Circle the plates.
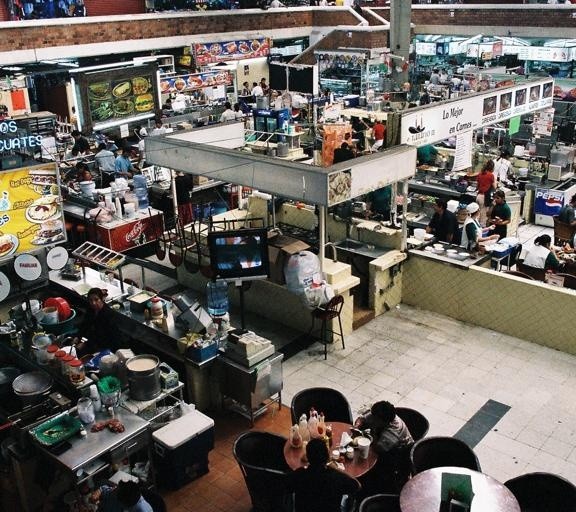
[188,78,202,87]
[446,249,458,254]
[0,271,11,303]
[12,253,43,281]
[26,201,61,223]
[239,44,250,54]
[47,247,68,271]
[458,252,470,257]
[174,78,186,92]
[114,101,134,114]
[250,42,261,51]
[113,80,131,98]
[0,234,19,260]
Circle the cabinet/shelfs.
[336,67,361,94]
[29,397,150,511]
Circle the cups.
[105,195,111,206]
[358,437,371,460]
[123,301,131,314]
[124,202,136,221]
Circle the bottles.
[338,448,346,460]
[308,408,318,438]
[115,197,122,218]
[63,353,76,377]
[77,397,95,423]
[151,297,164,323]
[316,413,325,437]
[289,424,302,448]
[298,413,309,441]
[321,435,329,453]
[332,450,339,460]
[326,426,334,447]
[47,345,61,367]
[144,309,149,321]
[346,447,354,460]
[161,316,168,333]
[89,384,102,412]
[54,350,64,371]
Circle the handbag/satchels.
[299,277,339,311]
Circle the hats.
[435,198,447,210]
[466,202,479,217]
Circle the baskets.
[34,414,82,444]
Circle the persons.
[344,133,357,153]
[136,123,152,169]
[93,144,115,188]
[426,199,459,243]
[71,130,90,157]
[117,136,127,149]
[561,230,576,254]
[162,98,173,110]
[175,169,193,226]
[333,142,355,164]
[348,401,416,494]
[371,119,385,153]
[191,88,199,105]
[461,202,501,250]
[352,119,370,152]
[60,162,97,201]
[472,159,497,228]
[269,88,283,110]
[514,142,526,158]
[489,150,515,187]
[70,288,122,353]
[241,81,250,95]
[217,102,236,122]
[232,102,244,119]
[486,190,510,241]
[250,83,264,103]
[521,235,559,282]
[0,104,9,121]
[287,438,362,512]
[106,140,118,151]
[150,119,173,136]
[427,68,490,92]
[232,237,261,269]
[192,88,209,105]
[98,480,153,511]
[259,78,269,95]
[113,148,139,180]
[468,57,491,68]
[366,185,391,221]
[398,83,419,102]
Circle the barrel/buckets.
[12,372,53,405]
[519,168,528,177]
[205,279,230,316]
[41,306,59,324]
[133,176,148,201]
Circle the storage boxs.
[152,409,215,469]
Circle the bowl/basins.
[434,248,445,254]
[414,229,426,241]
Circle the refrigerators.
[253,108,290,143]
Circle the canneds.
[47,345,58,369]
[62,355,75,376]
[54,351,66,373]
[150,297,163,318]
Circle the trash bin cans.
[152,409,215,491]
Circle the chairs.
[232,431,291,512]
[504,472,576,512]
[291,387,353,426]
[310,295,344,360]
[283,491,358,511]
[410,436,481,476]
[359,494,402,512]
[502,215,576,290]
[147,207,235,279]
[396,407,429,440]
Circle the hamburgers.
[134,93,154,113]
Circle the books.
[439,472,475,512]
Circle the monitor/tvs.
[207,228,271,287]
